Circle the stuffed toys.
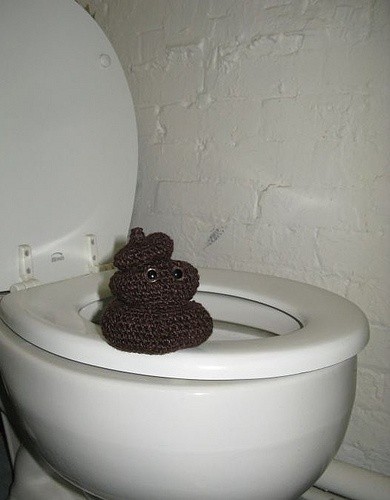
[102,224,214,357]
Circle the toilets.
[1,1,369,500]
